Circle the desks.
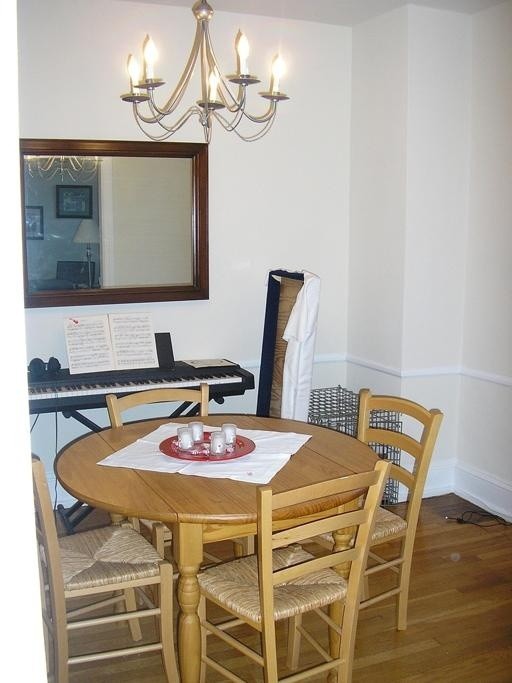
[54,413,384,683]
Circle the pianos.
[28,359,255,414]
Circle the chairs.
[197,458,393,683]
[296,388,443,630]
[105,381,256,581]
[31,452,182,683]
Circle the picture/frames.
[24,206,44,240]
[55,261,95,287]
[56,184,93,219]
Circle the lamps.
[120,2,289,144]
[24,155,101,184]
[72,219,100,289]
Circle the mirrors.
[19,137,210,309]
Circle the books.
[64,311,159,375]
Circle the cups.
[209,430,227,455]
[188,421,204,443]
[177,425,194,449]
[221,423,237,448]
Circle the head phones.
[28,356,61,378]
